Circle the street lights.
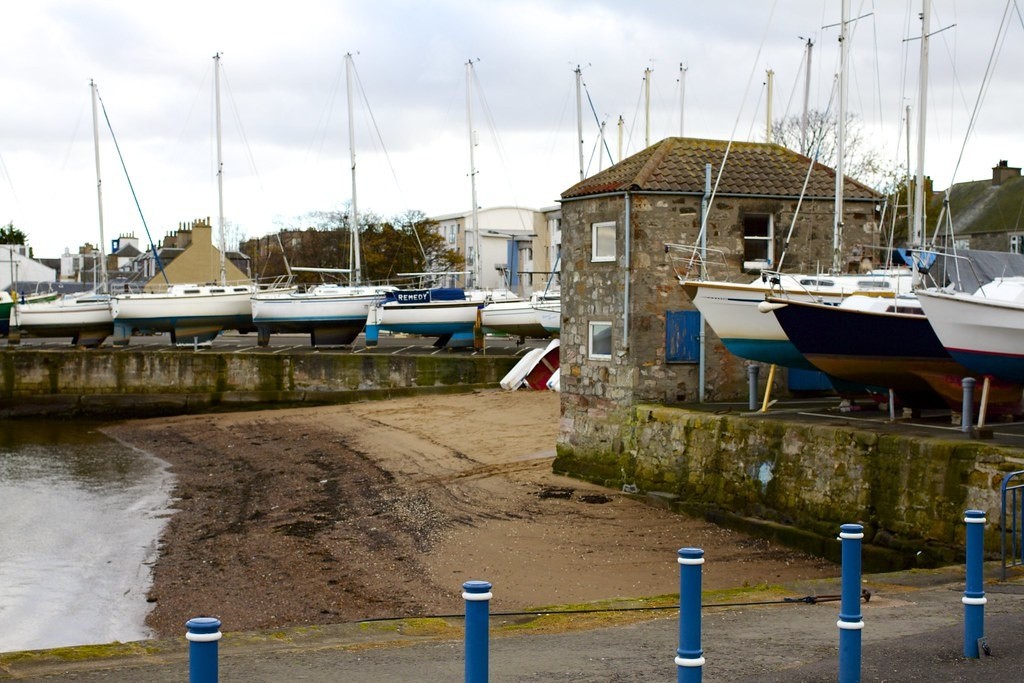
[488,230,538,291]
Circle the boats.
[500,338,560,392]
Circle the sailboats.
[109,51,297,347]
[663,0,1024,415]
[0,155,57,337]
[8,78,171,349]
[250,52,437,347]
[481,63,653,338]
[366,58,530,347]
